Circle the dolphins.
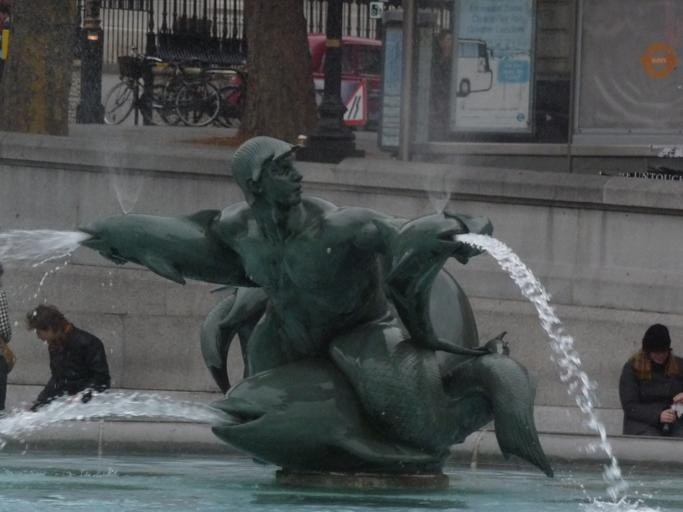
[78,212,264,289]
[209,360,494,467]
[384,212,512,355]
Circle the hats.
[235,32,383,127]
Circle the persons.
[24,305,111,410]
[619,324,683,436]
[0,267,12,416]
[211,135,403,373]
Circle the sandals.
[457,37,494,96]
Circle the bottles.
[641,324,674,353]
